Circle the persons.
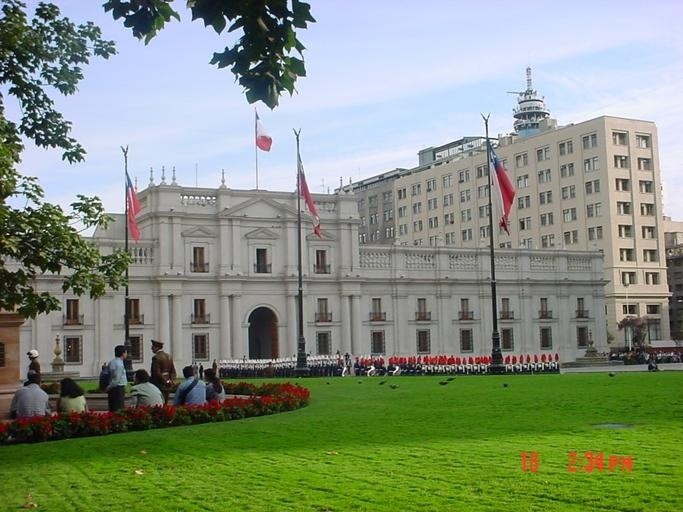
[26,349,39,381]
[150,338,181,407]
[212,360,217,376]
[131,369,165,410]
[10,373,51,419]
[191,361,195,374]
[56,377,88,421]
[612,349,682,363]
[23,369,36,387]
[199,363,204,379]
[194,362,199,380]
[105,344,129,413]
[202,368,225,403]
[174,366,207,406]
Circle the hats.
[151,339,163,347]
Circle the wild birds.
[380,379,386,385]
[503,383,508,387]
[438,381,448,385]
[357,380,363,384]
[447,377,456,381]
[609,372,615,377]
[389,383,399,388]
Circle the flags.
[290,126,323,241]
[479,110,515,239]
[120,144,142,248]
[254,108,273,154]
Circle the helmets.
[27,350,39,358]
